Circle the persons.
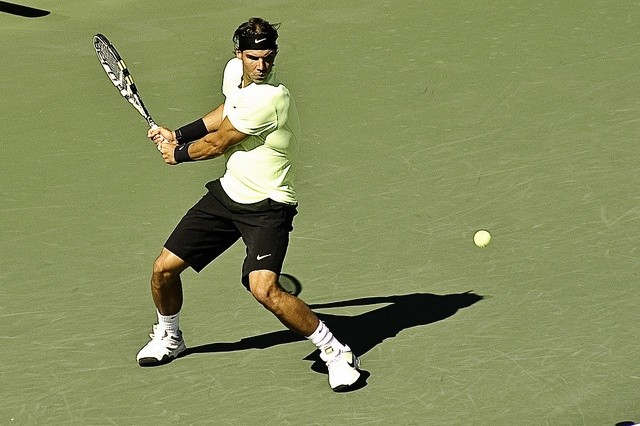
[135,16,363,392]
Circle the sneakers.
[319,344,361,392]
[136,325,188,367]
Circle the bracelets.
[175,118,209,141]
[172,142,194,167]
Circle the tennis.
[474,229,492,249]
